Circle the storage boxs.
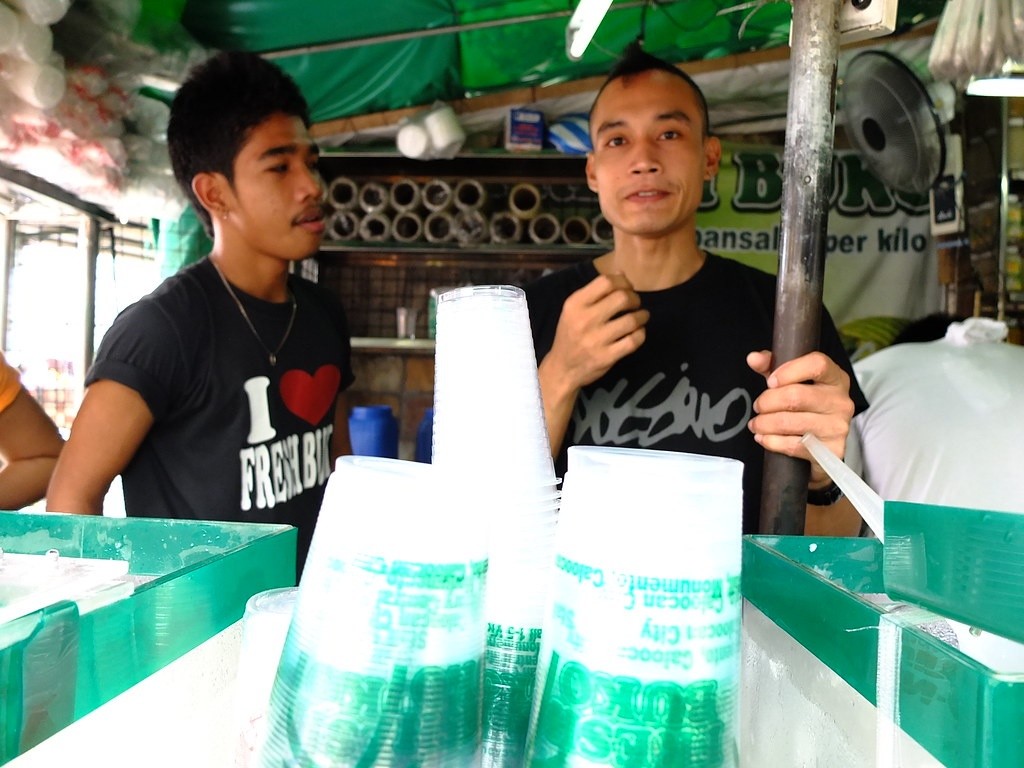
[741,499,1024,768]
[0,508,297,768]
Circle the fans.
[840,49,966,239]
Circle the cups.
[0,1,75,107]
[426,107,465,151]
[397,126,431,161]
[235,287,743,767]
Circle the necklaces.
[209,253,296,365]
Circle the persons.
[0,349,65,510]
[45,49,355,586]
[527,54,868,536]
[834,310,1024,531]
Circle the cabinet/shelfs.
[309,151,618,354]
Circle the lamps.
[564,0,615,62]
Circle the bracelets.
[806,459,845,506]
